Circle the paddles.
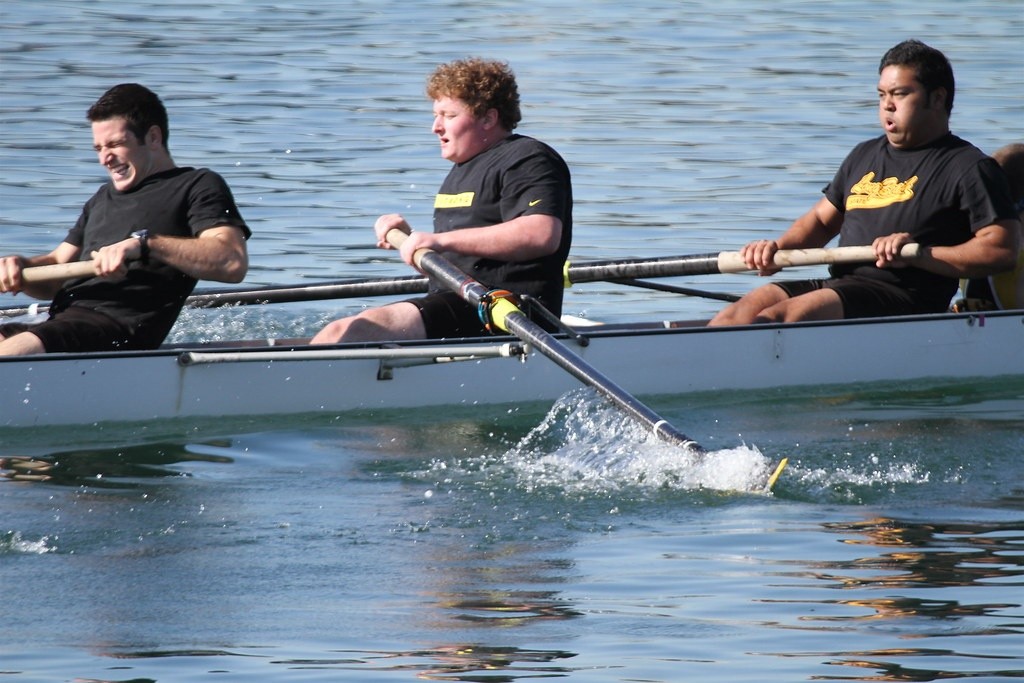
[4,258,97,284]
[0,243,920,322]
[384,225,790,494]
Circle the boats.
[1,296,1024,430]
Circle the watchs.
[131,229,149,260]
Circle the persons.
[0,83,252,359]
[309,56,572,350]
[704,38,1023,328]
